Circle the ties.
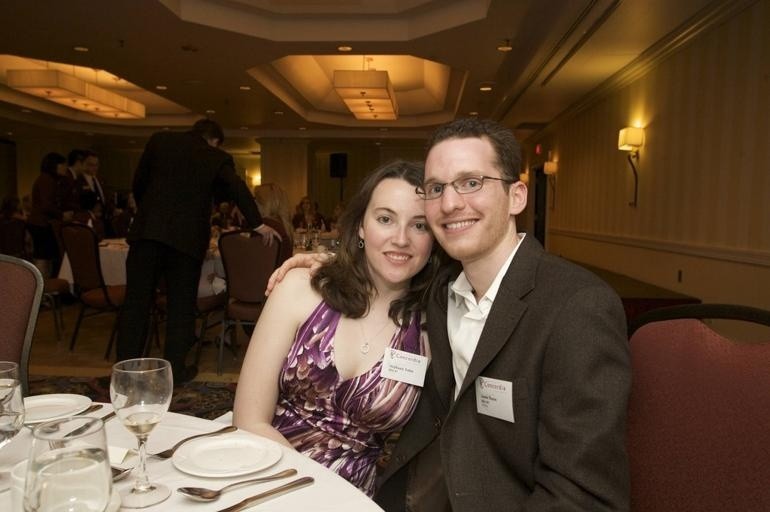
[91,177,103,207]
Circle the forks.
[131,425,239,461]
[46,438,68,450]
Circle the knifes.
[53,409,117,446]
[216,476,315,512]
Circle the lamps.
[618,127,647,207]
[334,55,401,121]
[5,61,146,120]
[543,161,561,212]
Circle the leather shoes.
[172,365,198,377]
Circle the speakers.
[327,151,347,178]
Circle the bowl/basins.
[8,459,25,510]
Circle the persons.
[113,190,127,230]
[231,157,450,497]
[259,114,635,511]
[291,197,326,235]
[2,195,27,224]
[125,192,138,238]
[240,183,295,270]
[210,202,237,230]
[63,147,90,237]
[112,116,284,388]
[78,152,122,243]
[320,201,349,241]
[27,152,74,310]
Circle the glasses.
[415,174,513,200]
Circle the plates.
[1,487,122,512]
[23,393,92,424]
[172,435,282,478]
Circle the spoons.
[113,468,135,482]
[175,468,298,503]
[36,404,103,434]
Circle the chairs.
[218,229,281,330]
[0,254,44,395]
[43,278,70,341]
[145,279,239,368]
[61,222,127,360]
[627,302,770,511]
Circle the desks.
[561,255,704,320]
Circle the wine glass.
[22,417,113,511]
[109,357,171,510]
[1,359,25,496]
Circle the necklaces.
[360,316,391,353]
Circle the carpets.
[29,374,237,422]
